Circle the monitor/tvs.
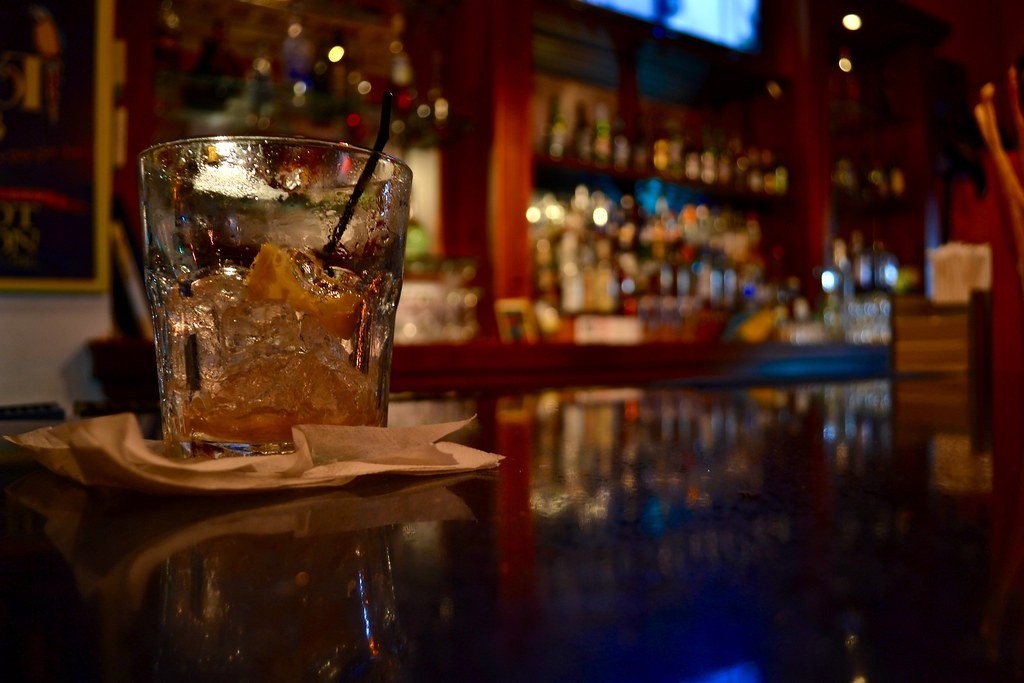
[571,0,768,61]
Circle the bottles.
[527,380,887,496]
[833,232,900,299]
[541,200,791,314]
[536,96,787,192]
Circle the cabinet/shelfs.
[442,0,930,338]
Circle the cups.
[158,526,402,683]
[140,136,416,462]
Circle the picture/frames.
[0,0,116,294]
